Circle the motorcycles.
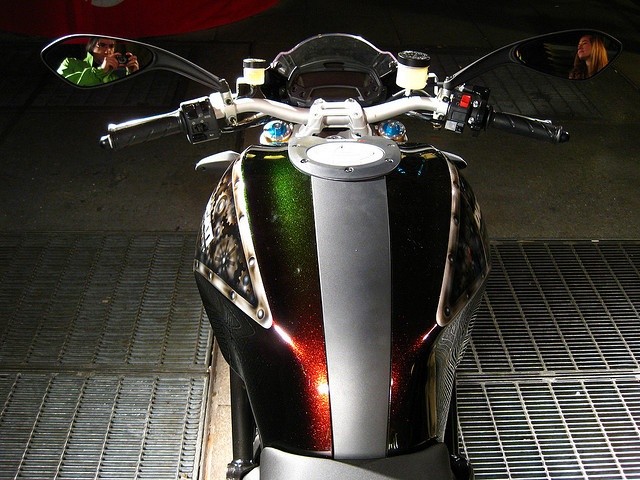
[37,27,625,480]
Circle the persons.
[56,36,140,88]
[571,33,609,81]
